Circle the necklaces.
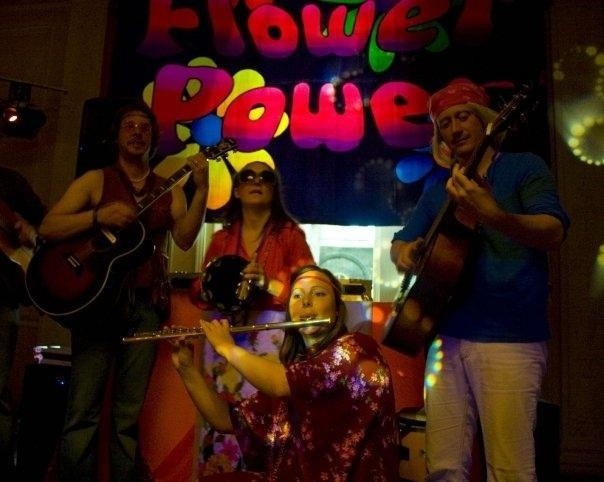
[114,167,150,183]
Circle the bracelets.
[92,206,97,226]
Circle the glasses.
[120,119,153,133]
[235,168,277,184]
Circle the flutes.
[121,318,332,344]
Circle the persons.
[199,160,315,482]
[162,265,401,482]
[390,77,570,482]
[39,109,208,482]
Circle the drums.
[397,404,483,482]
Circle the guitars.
[24,136,238,319]
[381,88,535,359]
